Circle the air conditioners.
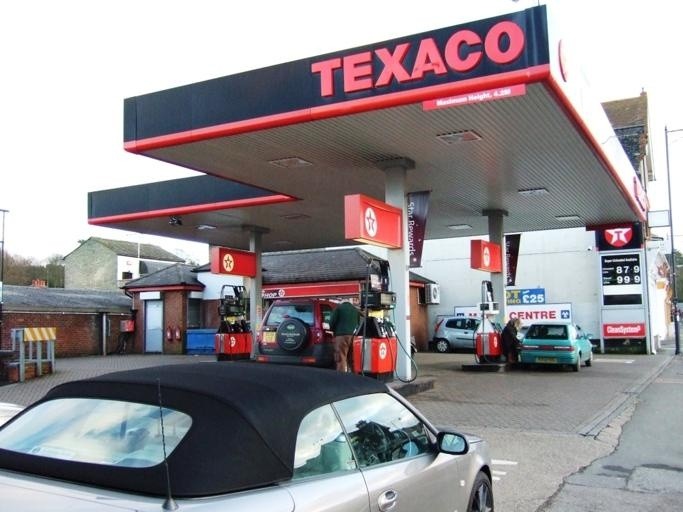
[423,283,441,304]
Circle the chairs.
[537,327,548,338]
[450,321,457,327]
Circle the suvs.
[252,298,365,368]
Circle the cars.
[0,362,495,511]
[433,317,496,353]
[517,322,595,372]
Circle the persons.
[499,318,521,362]
[328,297,361,373]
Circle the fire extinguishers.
[165,325,181,340]
